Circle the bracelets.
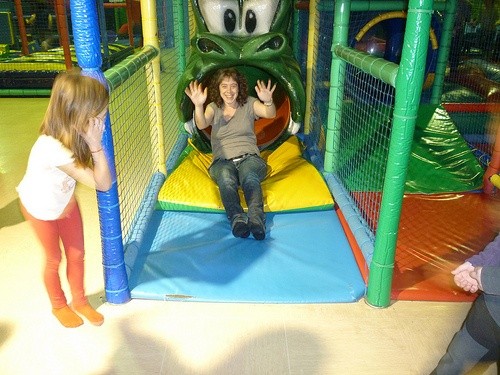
[91,149,102,154]
[264,98,272,105]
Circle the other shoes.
[231,217,250,238]
[248,214,265,241]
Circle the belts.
[229,155,242,160]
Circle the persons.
[184,68,276,240]
[26,36,40,52]
[119,20,135,37]
[430,234,500,375]
[17,73,112,327]
